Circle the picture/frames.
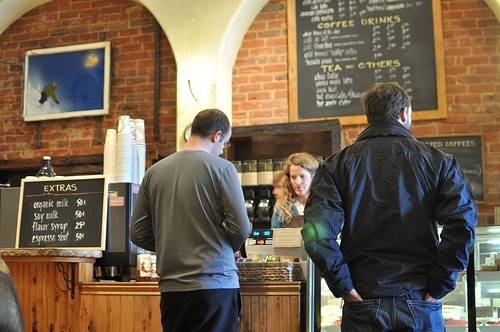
[23,41,111,122]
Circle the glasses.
[221,133,231,149]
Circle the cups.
[103,115,146,191]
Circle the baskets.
[236,261,302,282]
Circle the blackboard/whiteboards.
[414,133,486,204]
[286,0,449,126]
[14,174,110,250]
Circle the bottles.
[35,155,57,177]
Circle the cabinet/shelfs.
[314,224,500,332]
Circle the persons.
[271,152,319,229]
[39,82,61,105]
[300,82,478,332]
[130,109,250,332]
